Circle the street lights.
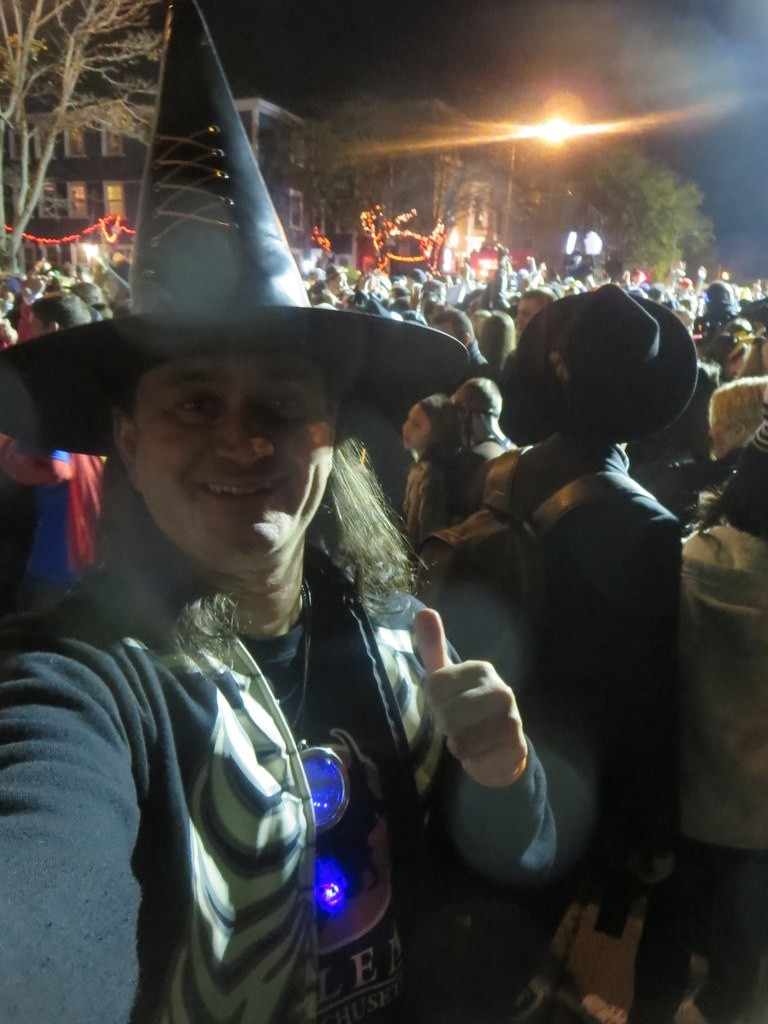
[503,112,578,242]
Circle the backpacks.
[415,447,658,702]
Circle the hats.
[407,265,425,284]
[0,0,469,456]
[515,284,698,443]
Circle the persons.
[0,245,768,661]
[676,419,768,1024]
[416,283,685,991]
[1,193,558,1024]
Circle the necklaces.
[268,582,351,832]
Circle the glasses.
[298,744,352,927]
